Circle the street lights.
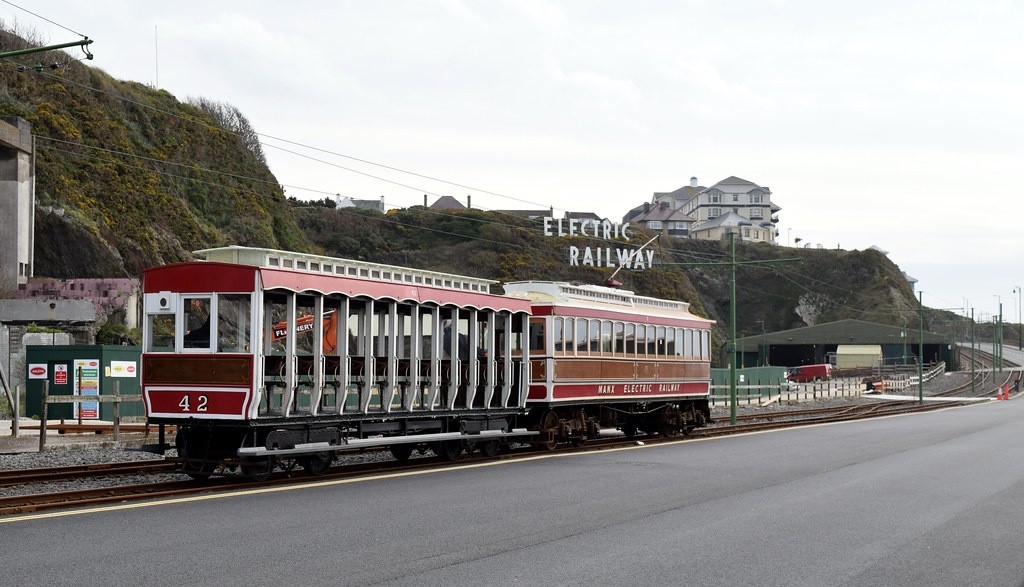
[788,227,791,247]
[728,221,732,232]
[1013,286,1022,350]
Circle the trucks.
[787,364,832,383]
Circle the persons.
[171,313,210,348]
[444,320,488,360]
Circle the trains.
[140,261,716,481]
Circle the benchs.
[259,355,522,413]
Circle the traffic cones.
[997,383,1009,400]
